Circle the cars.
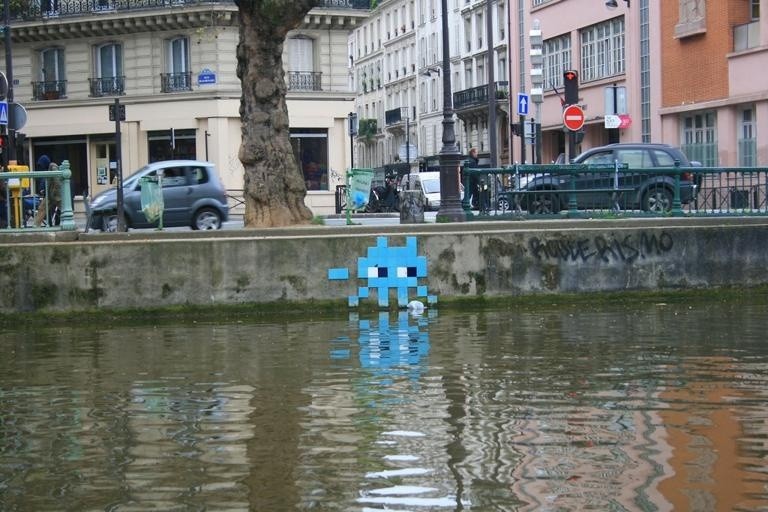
[497,144,703,214]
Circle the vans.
[88,160,229,232]
[399,170,473,210]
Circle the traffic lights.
[563,70,578,104]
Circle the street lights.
[529,19,543,163]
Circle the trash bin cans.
[351,166,376,211]
[138,180,164,226]
[731,190,748,209]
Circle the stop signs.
[563,105,584,131]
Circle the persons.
[462,148,480,211]
[38,153,63,227]
